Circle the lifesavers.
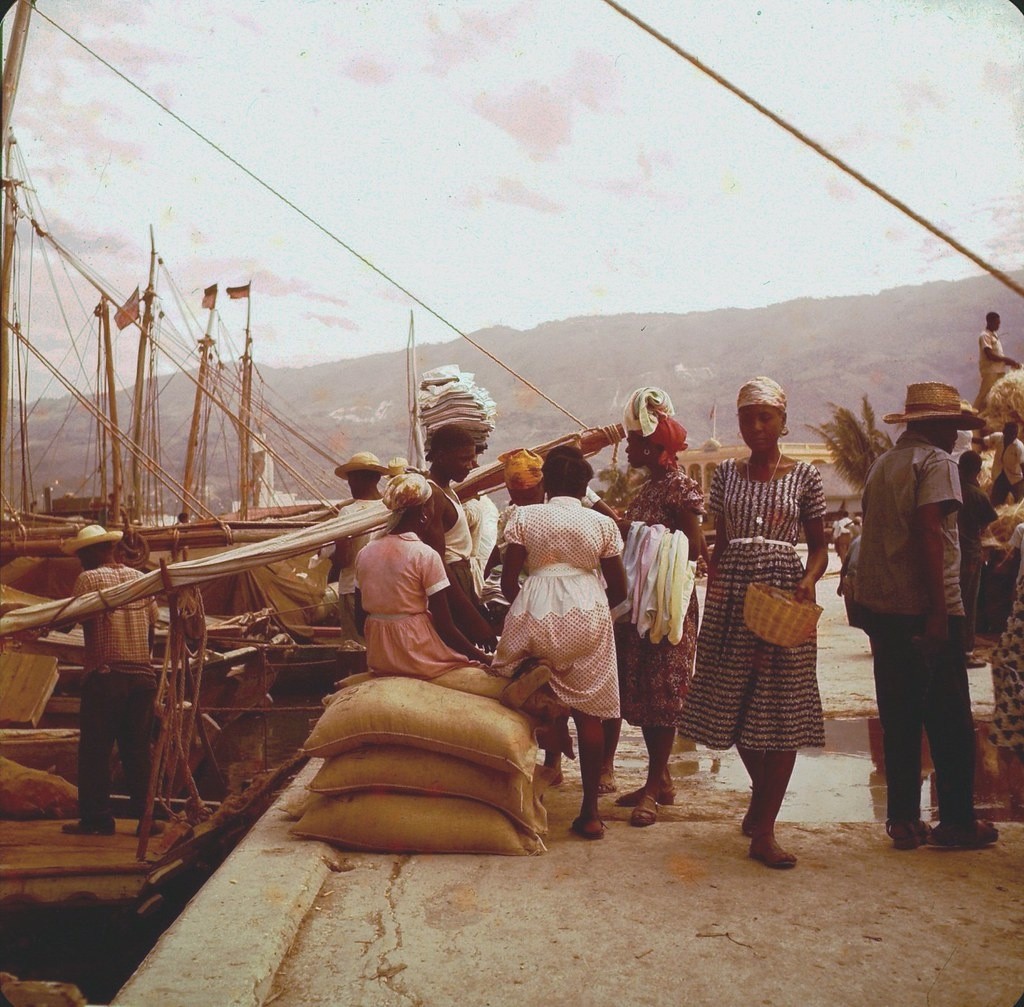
[1,893,38,911]
[62,891,97,909]
[115,532,150,569]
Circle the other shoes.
[63,822,114,835]
[500,657,552,710]
[965,655,986,668]
[749,847,796,867]
[136,822,165,838]
[743,789,760,837]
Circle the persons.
[675,376,829,870]
[837,311,1024,848]
[0,524,167,836]
[335,414,703,839]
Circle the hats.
[498,447,544,489]
[334,452,390,480]
[737,377,788,413]
[882,383,986,431]
[58,525,123,556]
[426,425,476,461]
[388,457,408,475]
[373,473,432,540]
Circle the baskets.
[744,583,824,647]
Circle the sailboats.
[0,0,373,1006]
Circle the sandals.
[886,818,932,850]
[927,820,998,847]
[597,768,616,793]
[631,794,657,824]
[573,816,604,840]
[615,786,677,805]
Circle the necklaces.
[746,455,781,524]
